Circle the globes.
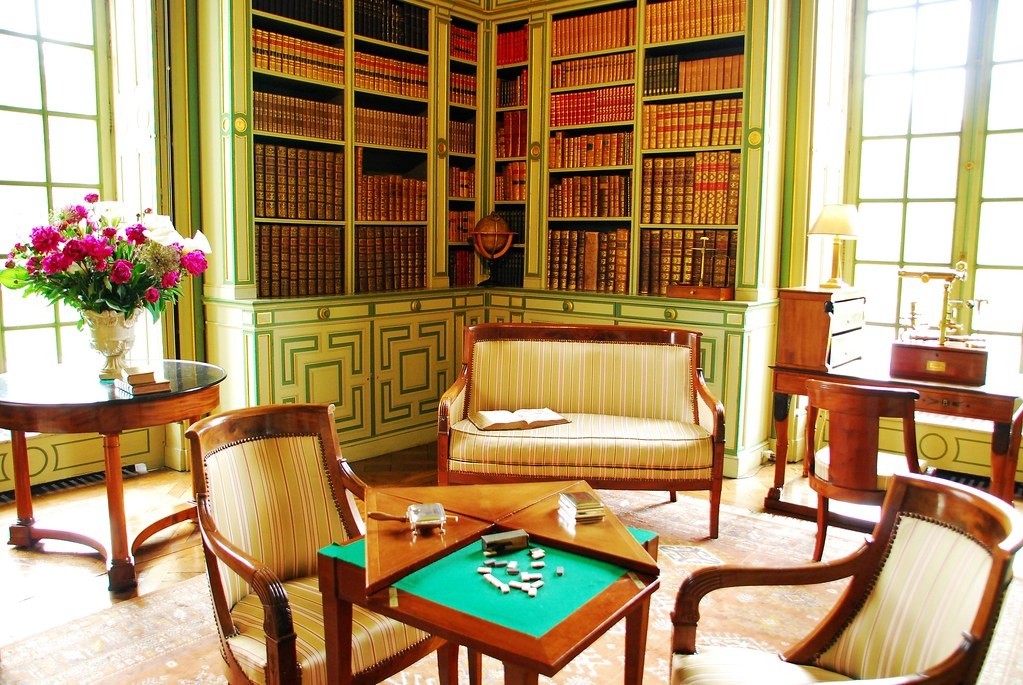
[464,210,520,288]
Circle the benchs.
[437,324,725,539]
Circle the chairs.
[184,403,461,685]
[806,378,938,562]
[669,473,1022,685]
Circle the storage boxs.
[780,284,866,371]
[890,340,988,386]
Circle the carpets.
[0,489,1023,685]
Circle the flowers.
[0,193,213,329]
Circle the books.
[556,491,605,525]
[468,406,571,430]
[113,365,171,395]
[249,0,748,303]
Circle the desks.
[314,479,660,685]
[768,364,1023,534]
[0,357,229,588]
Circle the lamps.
[898,268,966,345]
[808,204,866,290]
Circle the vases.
[82,307,140,382]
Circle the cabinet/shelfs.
[199,1,788,479]
